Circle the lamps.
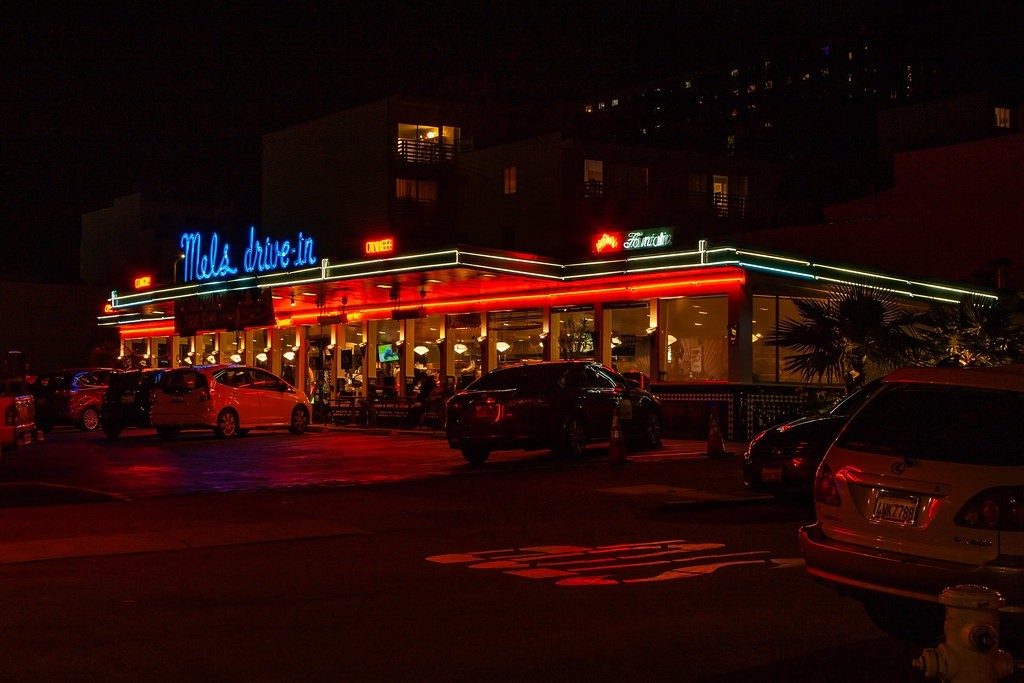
[255,330,268,362]
[290,297,296,306]
[496,314,510,353]
[414,319,430,355]
[230,341,241,363]
[282,328,295,361]
[454,329,468,355]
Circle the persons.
[407,372,438,429]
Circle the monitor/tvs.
[376,343,400,362]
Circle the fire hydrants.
[911,582,1018,683]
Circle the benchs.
[323,398,447,431]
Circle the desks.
[394,396,418,430]
[337,396,368,427]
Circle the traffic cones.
[602,407,634,466]
[699,404,737,459]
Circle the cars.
[741,374,883,513]
[150,362,313,441]
[0,346,166,452]
[445,360,663,465]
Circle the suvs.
[798,362,1023,631]
